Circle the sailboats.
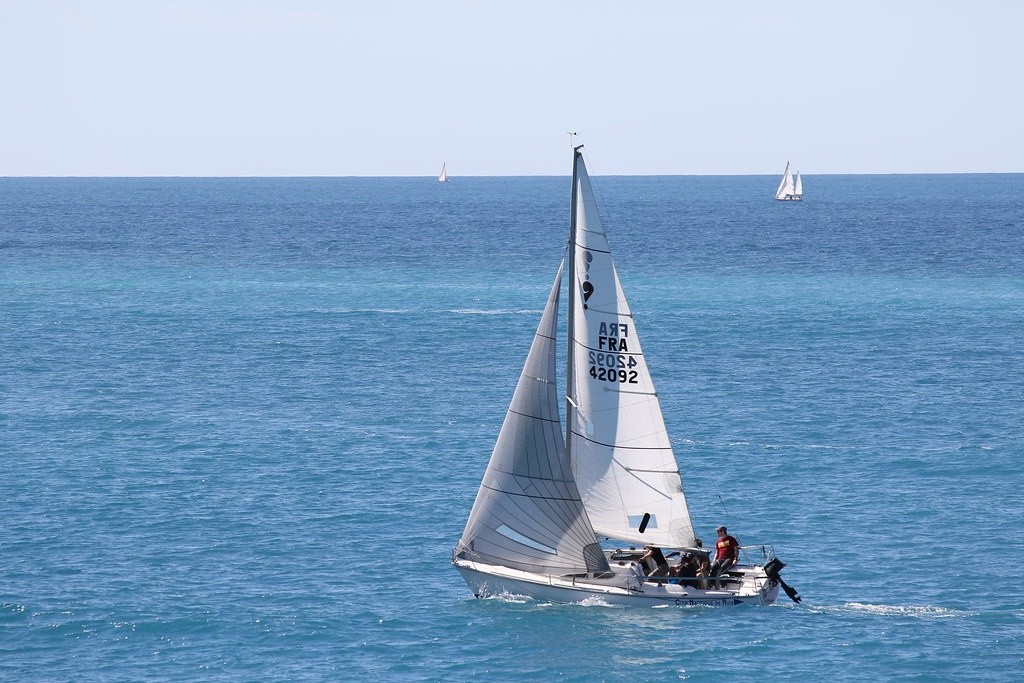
[438,163,449,182]
[451,130,778,607]
[774,161,803,200]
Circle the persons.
[708,526,738,590]
[640,545,669,582]
[679,539,710,589]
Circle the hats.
[716,525,728,532]
